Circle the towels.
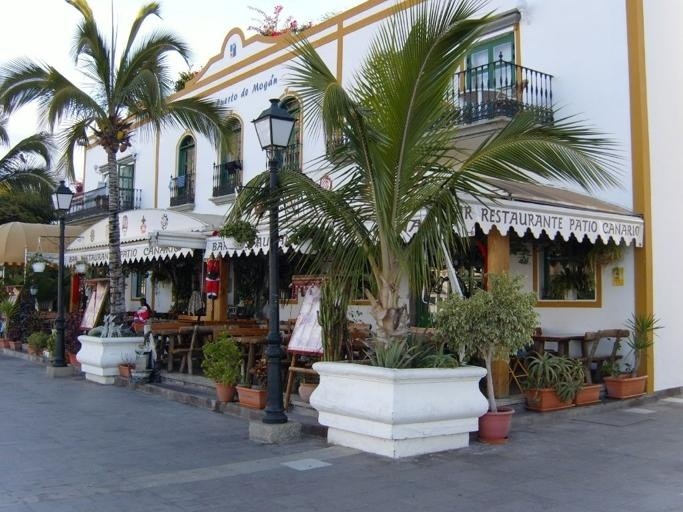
[177,175,186,188]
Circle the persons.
[132,298,152,322]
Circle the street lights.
[251,99,299,423]
[51,178,72,369]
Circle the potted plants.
[601,313,666,398]
[236,359,266,409]
[433,268,541,443]
[217,220,260,250]
[526,352,576,412]
[546,267,593,300]
[0,234,59,359]
[201,327,243,403]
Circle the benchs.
[574,329,630,384]
[144,320,372,388]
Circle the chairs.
[495,78,530,104]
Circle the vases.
[297,379,319,403]
[576,383,602,406]
[118,362,135,377]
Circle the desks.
[528,335,583,358]
[457,87,503,120]
